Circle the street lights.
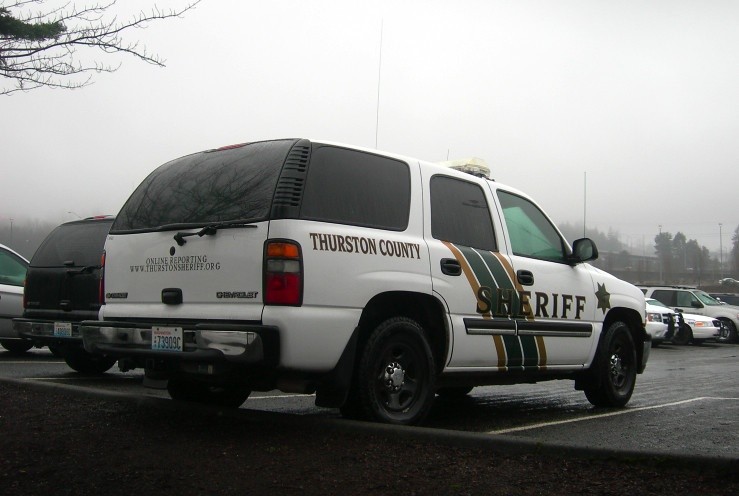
[718,222,723,293]
[657,224,663,282]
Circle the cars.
[719,277,739,285]
[634,283,739,349]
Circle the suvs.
[0,242,35,354]
[11,214,120,376]
[76,135,653,428]
